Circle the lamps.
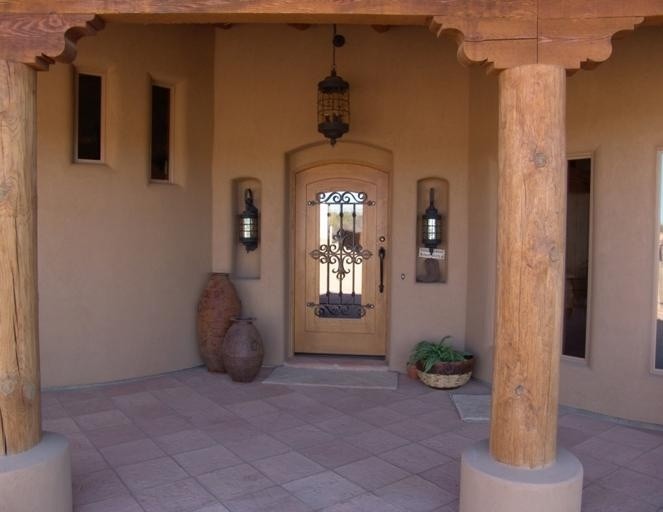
[316,23,352,147]
[237,188,259,251]
[421,187,443,254]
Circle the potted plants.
[406,338,478,390]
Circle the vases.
[198,273,264,383]
